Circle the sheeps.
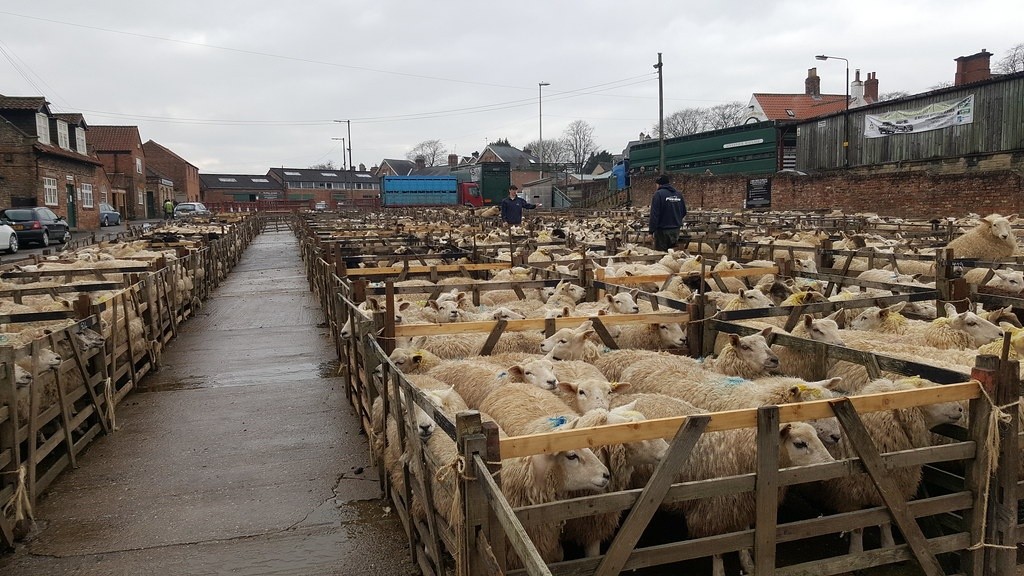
[130,212,252,285]
[306,207,626,287]
[0,242,214,505]
[341,293,1024,576]
[626,204,1023,329]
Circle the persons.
[501,185,541,228]
[648,174,688,252]
[163,200,173,219]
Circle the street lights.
[815,55,849,167]
[332,137,347,170]
[538,82,550,179]
[334,119,352,170]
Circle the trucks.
[379,175,484,209]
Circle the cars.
[174,203,212,220]
[99,204,121,227]
[315,202,327,211]
[0,219,19,253]
[0,206,72,248]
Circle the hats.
[655,175,669,185]
[509,185,518,190]
